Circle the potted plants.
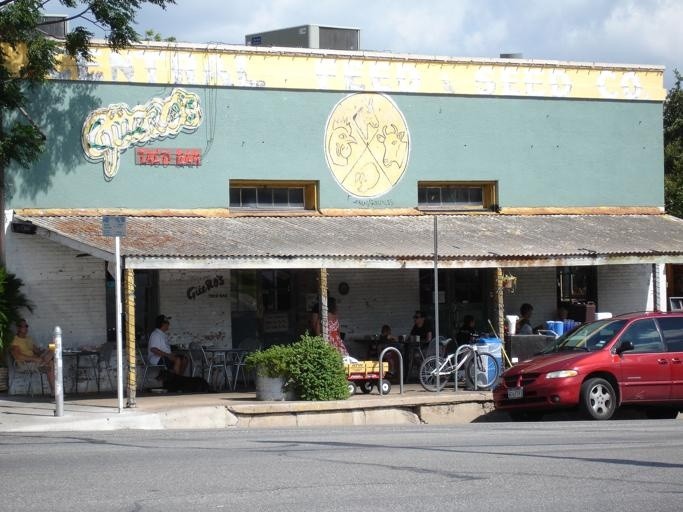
[242,329,352,401]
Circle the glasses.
[412,315,423,318]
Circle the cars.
[492,310,682,420]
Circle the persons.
[148,315,188,393]
[519,303,542,334]
[456,315,477,347]
[10,319,67,398]
[380,325,398,376]
[311,290,350,356]
[404,311,435,380]
[560,304,575,321]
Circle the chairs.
[4,327,446,397]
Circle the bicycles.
[418,326,498,392]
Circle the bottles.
[563,319,579,333]
[564,295,570,304]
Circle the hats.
[156,314,172,323]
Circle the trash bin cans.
[478,337,502,389]
[554,320,564,336]
[543,320,554,335]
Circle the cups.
[416,335,420,343]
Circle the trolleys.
[341,360,391,395]
